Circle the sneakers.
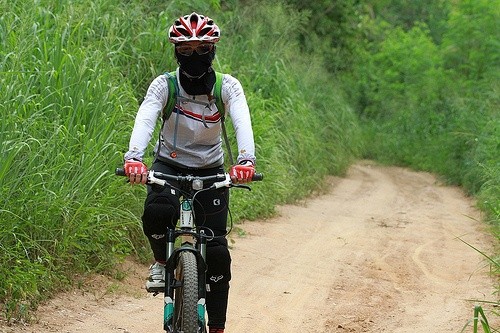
[144,262,167,293]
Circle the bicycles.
[114,167,264,333]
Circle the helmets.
[167,11,221,44]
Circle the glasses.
[175,43,214,55]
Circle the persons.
[124,12,256,333]
